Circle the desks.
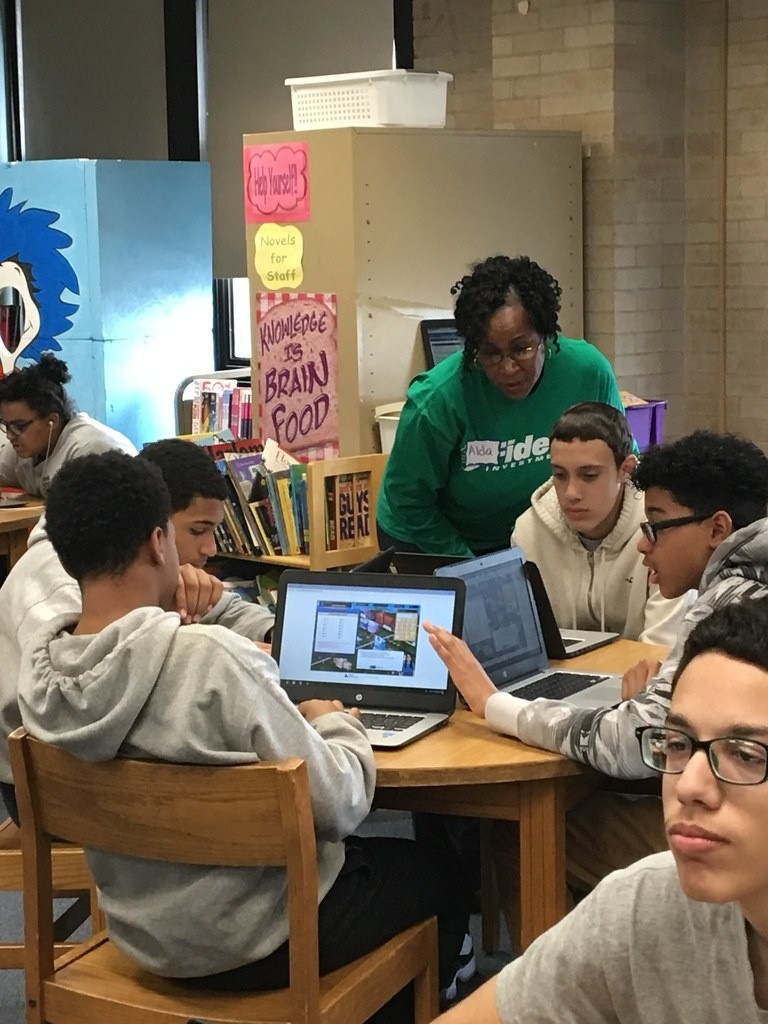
[372,638,676,956]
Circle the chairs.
[0,725,438,1024]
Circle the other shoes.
[440,927,476,999]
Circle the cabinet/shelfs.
[179,430,391,573]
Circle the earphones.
[49,420,54,426]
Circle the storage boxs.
[284,68,453,131]
[626,399,668,455]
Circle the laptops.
[272,547,623,750]
[420,319,465,371]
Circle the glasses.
[640,508,739,543]
[635,726,768,785]
[0,410,61,437]
[474,334,544,366]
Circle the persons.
[0,355,138,497]
[429,597,768,1024]
[376,255,637,574]
[18,449,476,1024]
[422,430,768,955]
[0,440,276,842]
[511,400,687,647]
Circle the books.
[179,378,369,558]
